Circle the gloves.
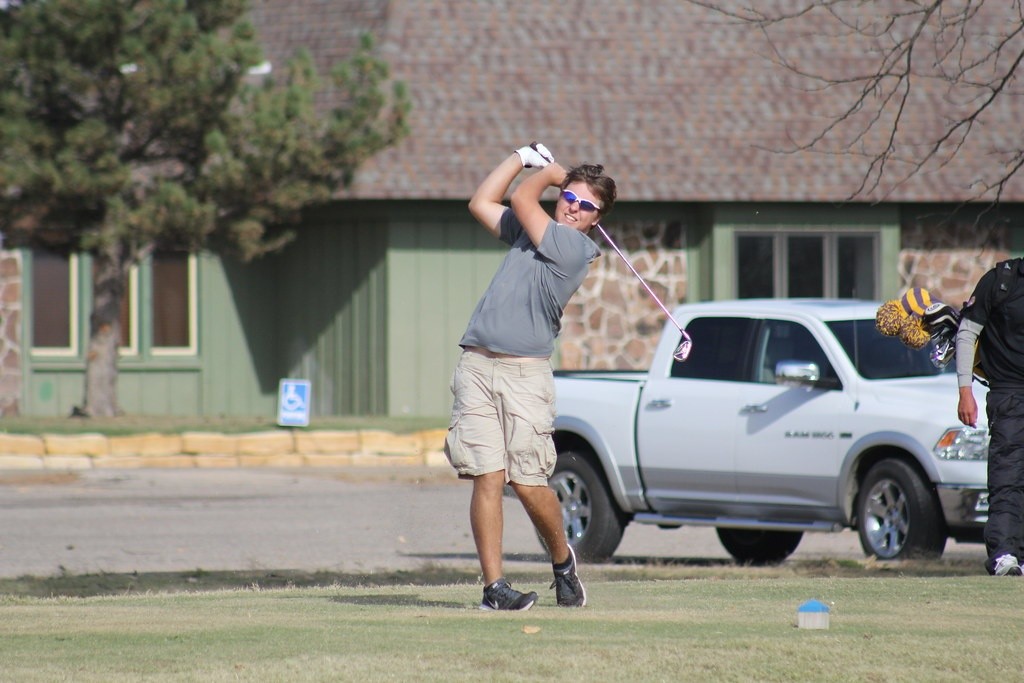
[514,143,555,168]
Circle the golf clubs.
[529,140,694,363]
[923,302,961,371]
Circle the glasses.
[562,190,601,212]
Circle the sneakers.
[992,551,1023,576]
[479,577,538,611]
[550,544,586,606]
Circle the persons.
[444,144,616,610]
[954,258,1024,577]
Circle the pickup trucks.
[535,297,992,564]
